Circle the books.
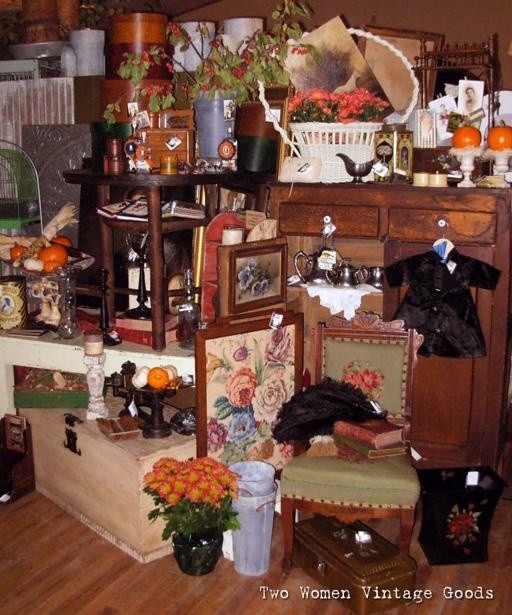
[334,417,408,460]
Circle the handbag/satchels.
[414,461,507,567]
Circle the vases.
[170,531,223,577]
[185,82,235,161]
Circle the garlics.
[160,363,177,380]
[24,254,43,272]
[131,366,149,387]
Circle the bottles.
[178,268,200,349]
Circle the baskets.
[252,28,419,185]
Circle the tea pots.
[294,248,384,290]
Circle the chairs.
[282,319,418,573]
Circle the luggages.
[291,511,418,614]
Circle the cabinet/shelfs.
[271,184,507,474]
[65,169,227,350]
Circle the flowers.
[102,0,316,124]
[141,452,248,538]
[286,83,389,125]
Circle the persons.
[465,87,477,113]
[439,104,448,117]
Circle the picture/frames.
[357,23,447,114]
[229,244,287,310]
[195,313,304,477]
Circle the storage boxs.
[17,403,196,564]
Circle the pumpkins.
[9,240,27,262]
[490,121,512,151]
[39,240,67,271]
[51,234,73,249]
[147,367,168,388]
[452,126,482,148]
[44,261,60,272]
